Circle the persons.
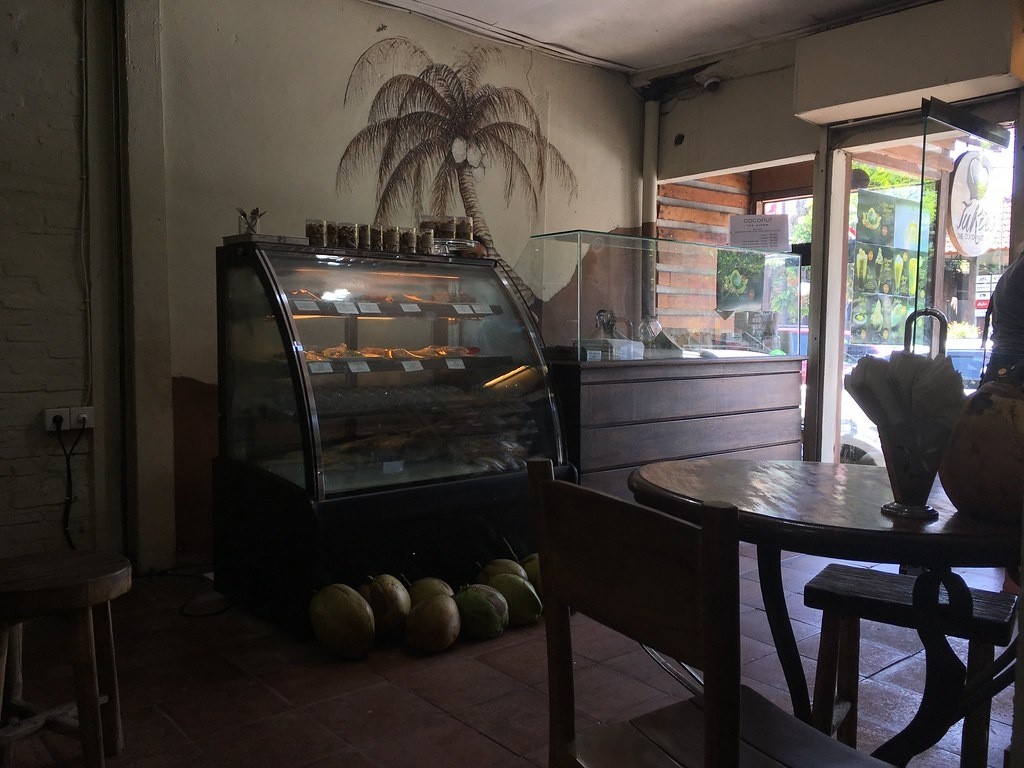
[979,253,1024,598]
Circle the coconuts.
[937,381,1024,523]
[308,553,542,646]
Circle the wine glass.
[875,259,883,293]
[871,314,883,343]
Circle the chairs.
[526,457,899,768]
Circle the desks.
[627,461,1024,768]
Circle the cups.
[909,258,918,297]
[853,313,868,340]
[890,311,902,343]
[895,258,904,294]
[857,253,868,292]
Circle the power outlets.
[44,407,70,432]
[70,406,95,430]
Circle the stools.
[804,565,1018,768]
[1,548,132,768]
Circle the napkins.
[843,352,965,426]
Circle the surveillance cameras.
[704,77,724,94]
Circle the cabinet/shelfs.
[528,229,812,506]
[213,241,580,655]
